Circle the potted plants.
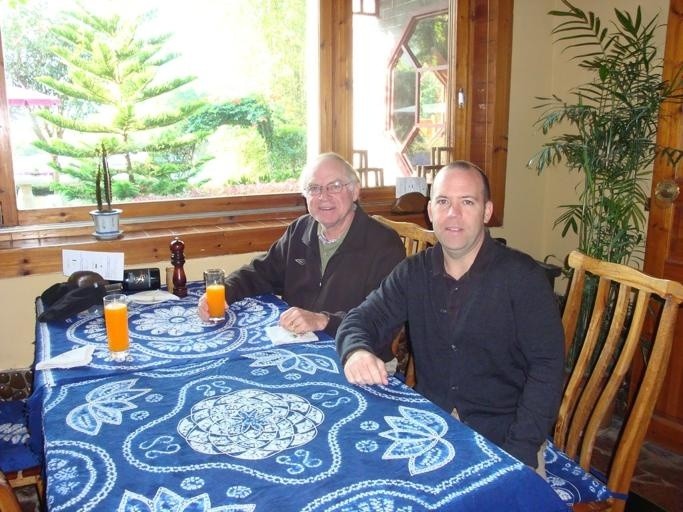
[88,142,124,234]
[527,1,683,432]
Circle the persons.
[335,160,564,472]
[198,153,406,377]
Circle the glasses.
[303,179,352,196]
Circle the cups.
[103,293,130,351]
[203,268,226,323]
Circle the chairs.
[365,211,438,390]
[541,248,683,512]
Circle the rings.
[290,321,293,326]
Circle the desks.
[36,279,577,512]
